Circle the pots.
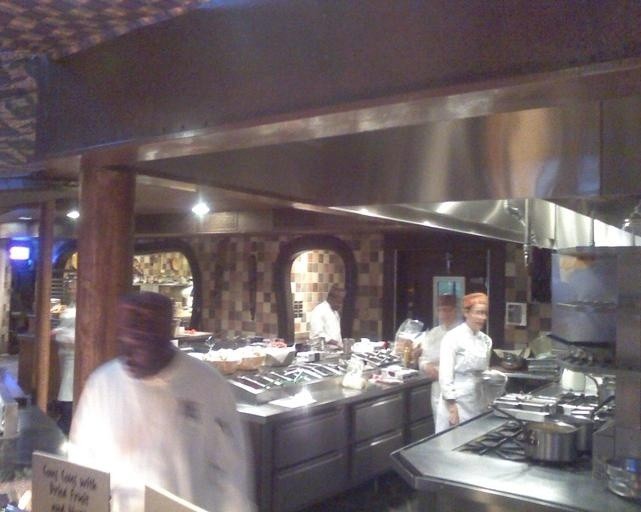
[524,422,577,461]
[607,457,641,499]
[544,416,593,451]
[546,333,611,362]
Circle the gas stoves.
[455,420,584,468]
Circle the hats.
[330,283,347,299]
[115,291,173,336]
[463,293,489,308]
[437,295,456,306]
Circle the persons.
[419,293,462,429]
[49,283,80,436]
[305,279,349,351]
[431,293,508,436]
[65,290,253,512]
[180,276,193,308]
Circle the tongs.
[283,368,305,383]
